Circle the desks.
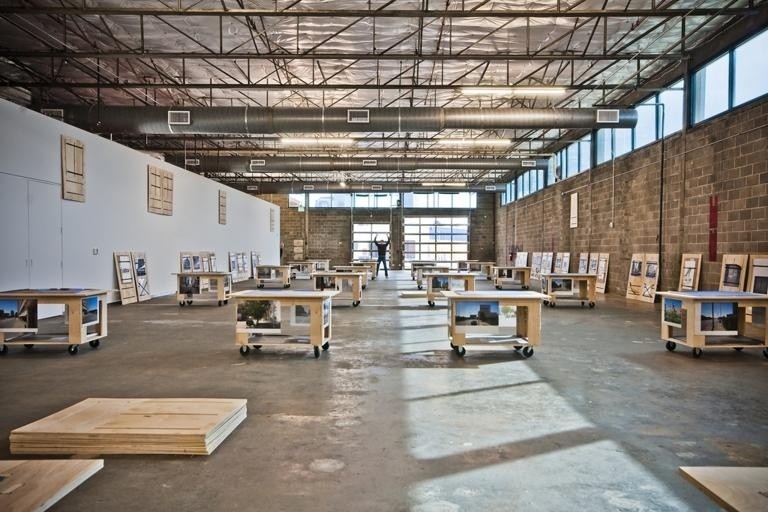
[255,265,291,289]
[174,272,233,305]
[349,261,379,280]
[333,265,371,289]
[307,259,331,272]
[465,261,496,280]
[230,290,342,358]
[541,273,602,309]
[679,465,768,512]
[0,287,118,355]
[287,261,315,280]
[0,459,104,512]
[490,266,532,290]
[411,262,436,280]
[423,272,479,308]
[415,265,449,290]
[656,290,768,361]
[457,260,479,272]
[441,289,552,358]
[310,273,367,307]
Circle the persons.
[374,234,390,277]
